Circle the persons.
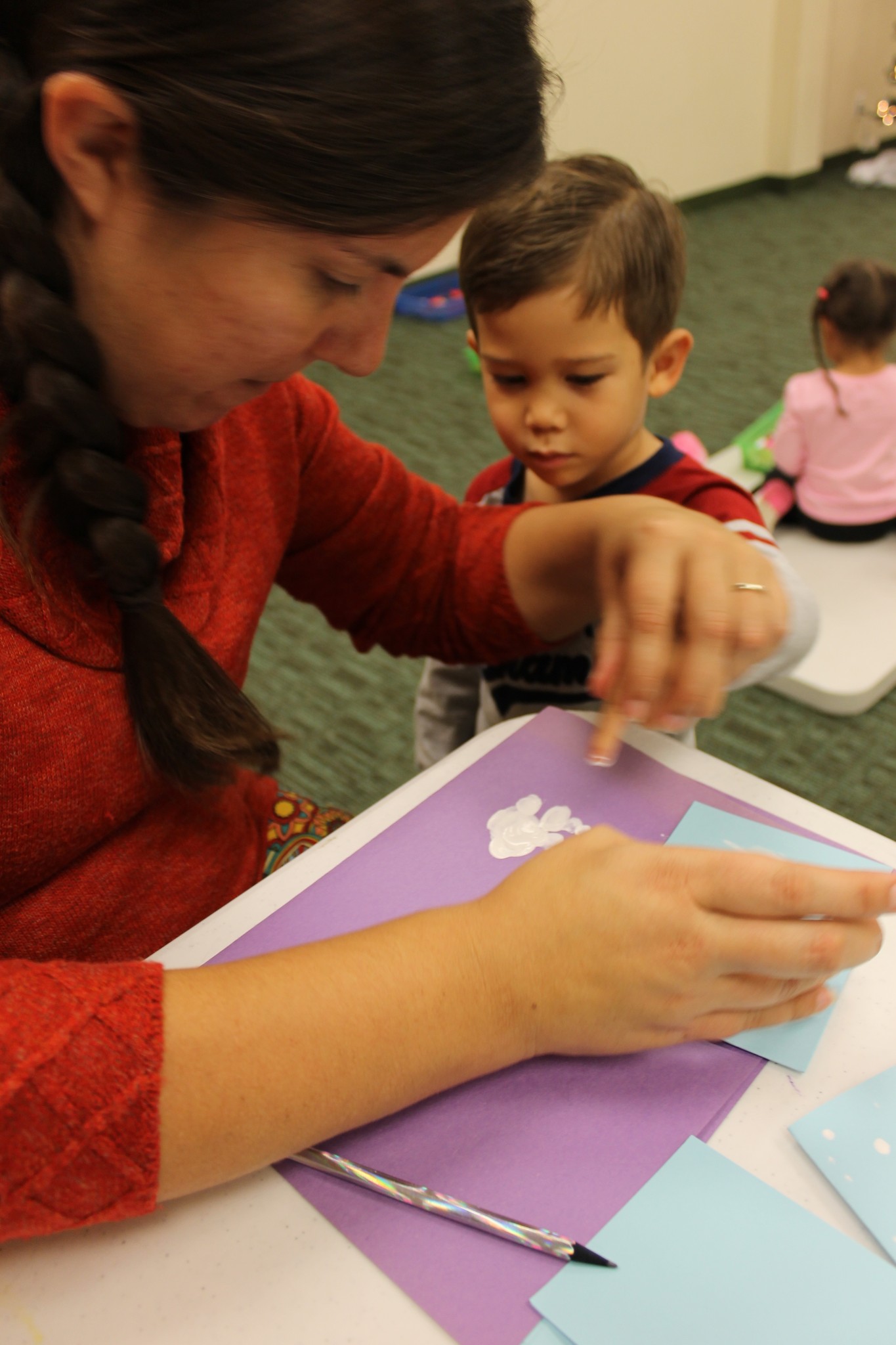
[411,152,818,754]
[1,1,896,1236]
[753,249,894,544]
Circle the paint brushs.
[286,1148,621,1275]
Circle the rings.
[733,581,768,592]
